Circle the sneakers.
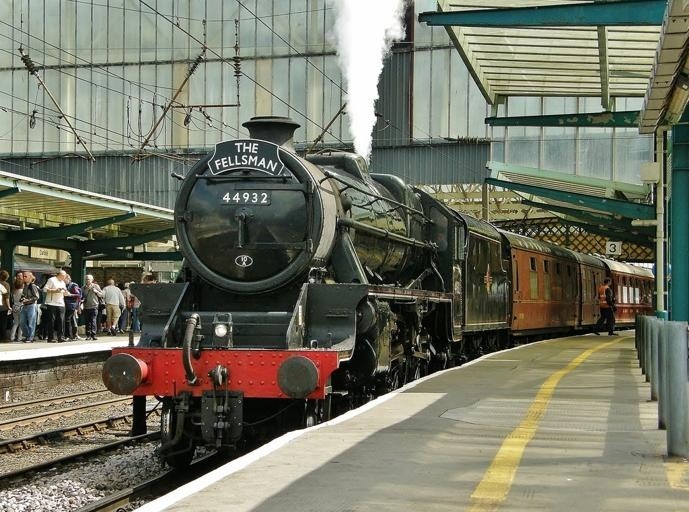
[608,332,617,336]
[9,335,80,343]
[593,329,600,336]
[86,334,97,340]
[98,327,125,335]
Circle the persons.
[0,268,159,343]
[593,278,620,336]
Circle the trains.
[101,113,655,464]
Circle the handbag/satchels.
[98,296,105,308]
[126,297,134,309]
[29,283,44,304]
